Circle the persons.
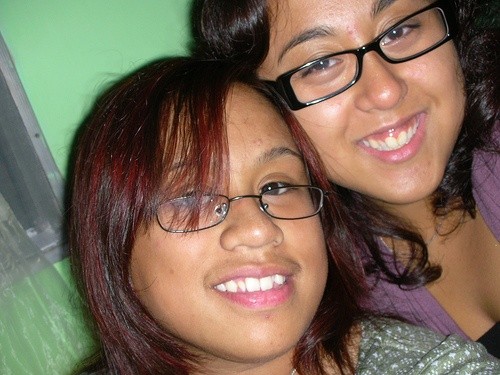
[69,56,500,375]
[191,0,500,361]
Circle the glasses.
[262,1,459,111]
[151,185,324,233]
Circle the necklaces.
[381,202,444,255]
[290,366,299,375]
